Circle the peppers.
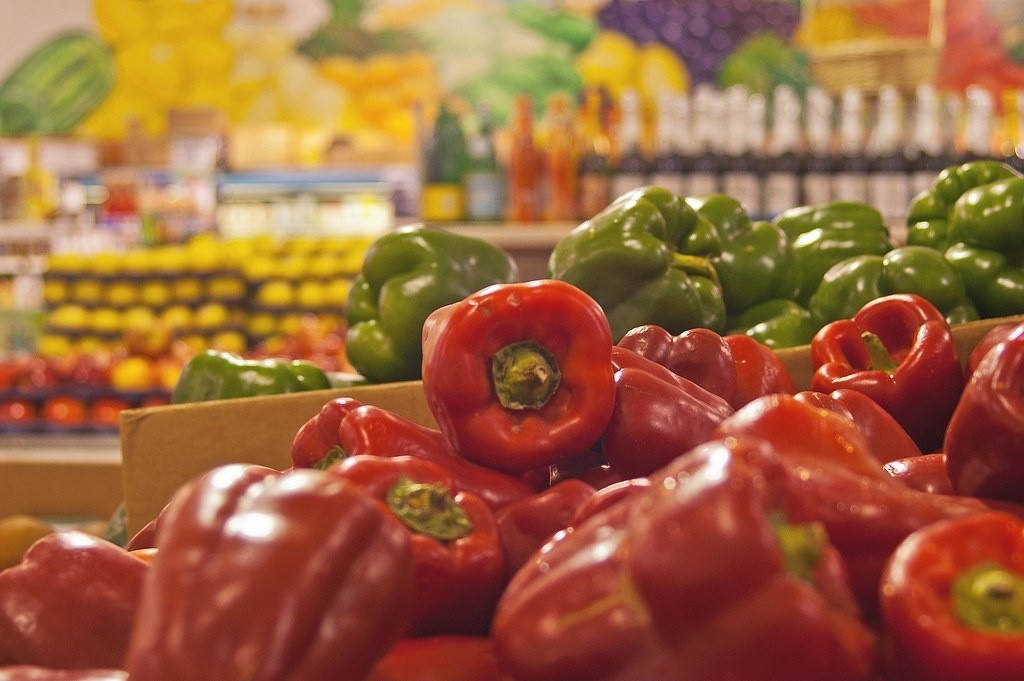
[0,159,1024,681]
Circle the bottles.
[417,83,1024,224]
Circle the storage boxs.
[0,310,1024,556]
